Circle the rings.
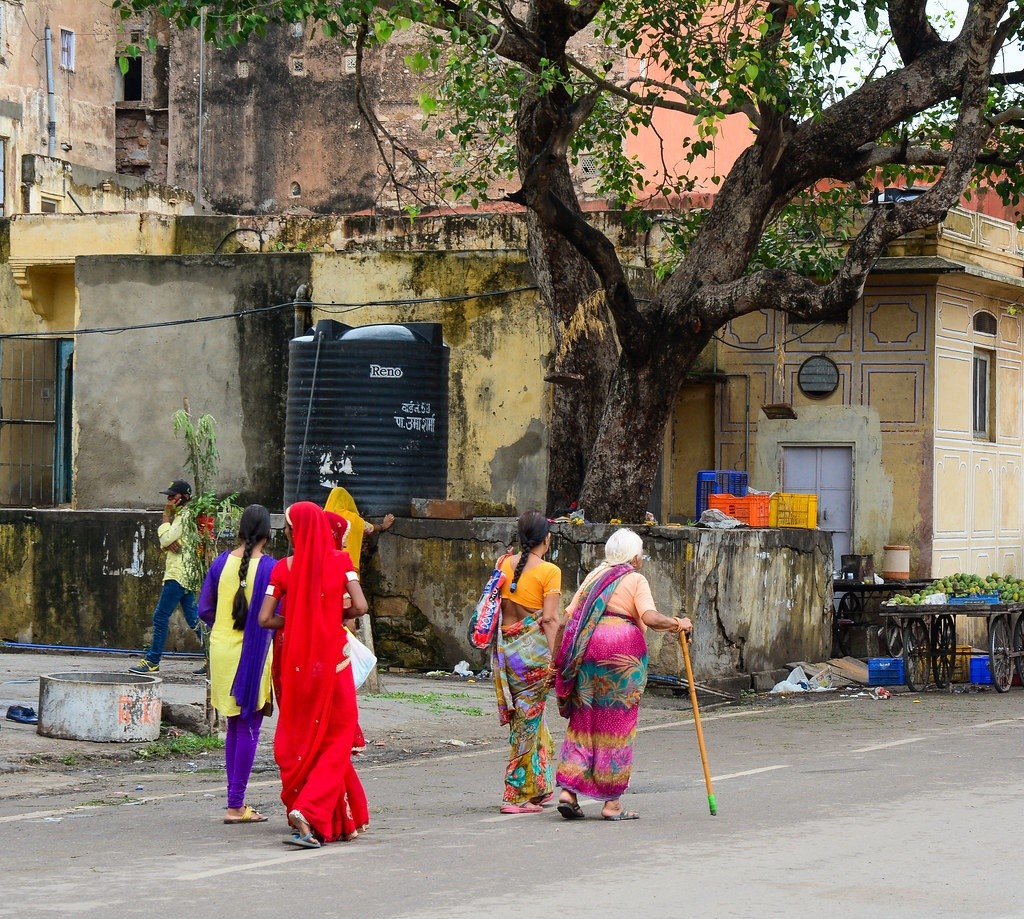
[391,517,394,519]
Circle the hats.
[159,479,192,496]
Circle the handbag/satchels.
[466,554,513,649]
[340,624,377,690]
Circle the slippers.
[557,801,584,819]
[223,804,268,823]
[531,792,554,805]
[500,801,543,812]
[282,831,322,848]
[605,808,640,821]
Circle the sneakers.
[192,659,207,676]
[128,660,160,674]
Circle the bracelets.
[546,666,558,676]
[672,616,682,633]
[378,524,383,530]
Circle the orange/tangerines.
[892,572,1024,607]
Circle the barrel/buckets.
[881,544,911,579]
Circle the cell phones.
[176,497,186,507]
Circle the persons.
[465,508,561,814]
[258,501,370,845]
[125,478,207,675]
[322,486,397,585]
[544,527,693,822]
[194,504,281,824]
[270,511,366,757]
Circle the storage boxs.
[767,492,819,529]
[866,656,907,686]
[694,469,748,525]
[913,644,973,684]
[706,493,770,529]
[947,591,1000,605]
[969,655,996,684]
